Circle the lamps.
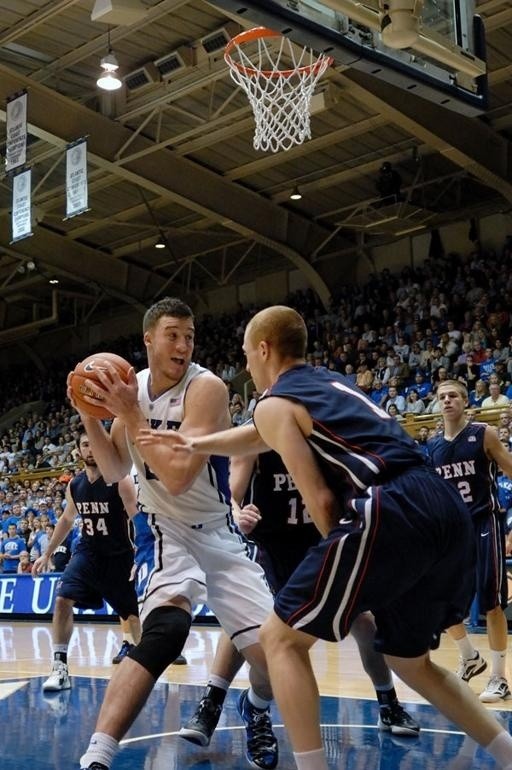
[95,23,122,92]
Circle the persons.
[216,356,235,378]
[64,433,76,446]
[229,351,241,370]
[407,369,432,400]
[71,425,79,437]
[498,427,509,439]
[436,420,444,429]
[180,416,420,746]
[466,410,476,422]
[356,363,372,391]
[236,320,246,335]
[205,356,216,374]
[390,355,408,384]
[422,379,447,420]
[499,440,511,453]
[370,379,387,403]
[66,455,71,463]
[432,367,447,392]
[71,522,84,558]
[458,355,479,390]
[113,511,186,665]
[0,375,69,575]
[224,379,238,400]
[385,387,404,411]
[380,376,402,408]
[402,390,425,416]
[490,373,500,385]
[508,418,512,431]
[345,364,356,382]
[136,305,512,769]
[233,393,242,405]
[493,468,512,513]
[468,380,484,408]
[375,358,389,384]
[75,468,81,476]
[479,347,497,380]
[232,403,245,424]
[74,454,81,464]
[428,381,512,703]
[29,425,140,694]
[418,426,428,445]
[305,259,509,368]
[481,383,507,413]
[388,404,401,419]
[499,412,510,426]
[248,390,259,409]
[494,359,509,387]
[228,402,234,415]
[66,298,278,769]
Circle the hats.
[494,359,501,365]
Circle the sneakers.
[113,641,129,664]
[478,676,509,702]
[172,653,186,665]
[455,650,487,681]
[179,698,222,747]
[236,688,279,770]
[377,703,420,736]
[43,660,71,690]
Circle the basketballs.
[68,352,132,422]
